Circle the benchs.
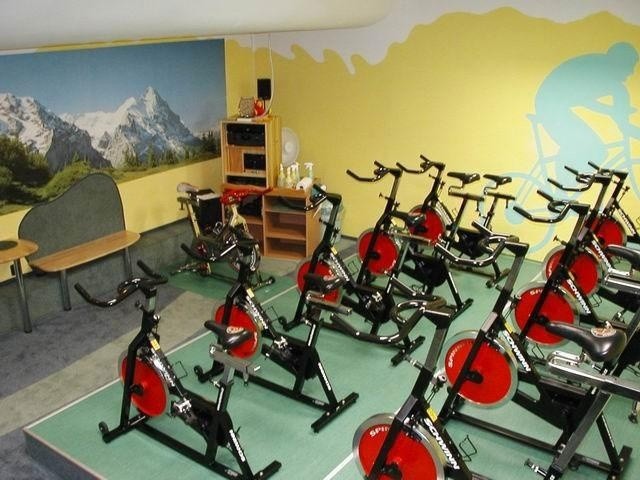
[17,172,141,311]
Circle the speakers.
[257,79,271,100]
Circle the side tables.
[0,238,39,333]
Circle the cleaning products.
[277,161,313,188]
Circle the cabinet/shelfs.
[220,117,322,261]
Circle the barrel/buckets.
[321,199,344,243]
